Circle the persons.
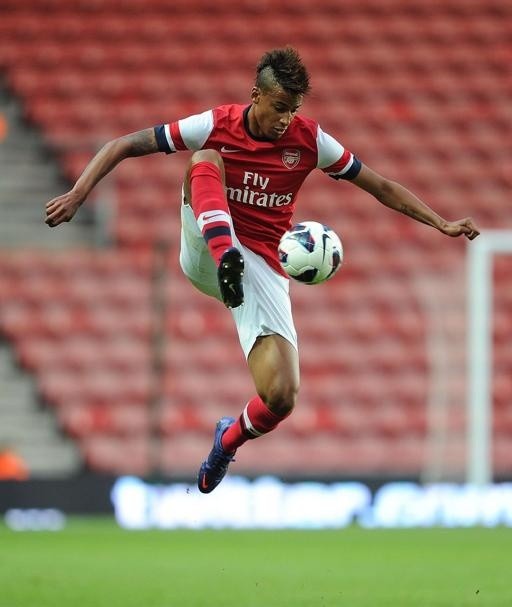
[43,47,480,494]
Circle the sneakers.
[198,417,237,492]
[217,247,244,308]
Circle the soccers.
[278,220,344,283]
